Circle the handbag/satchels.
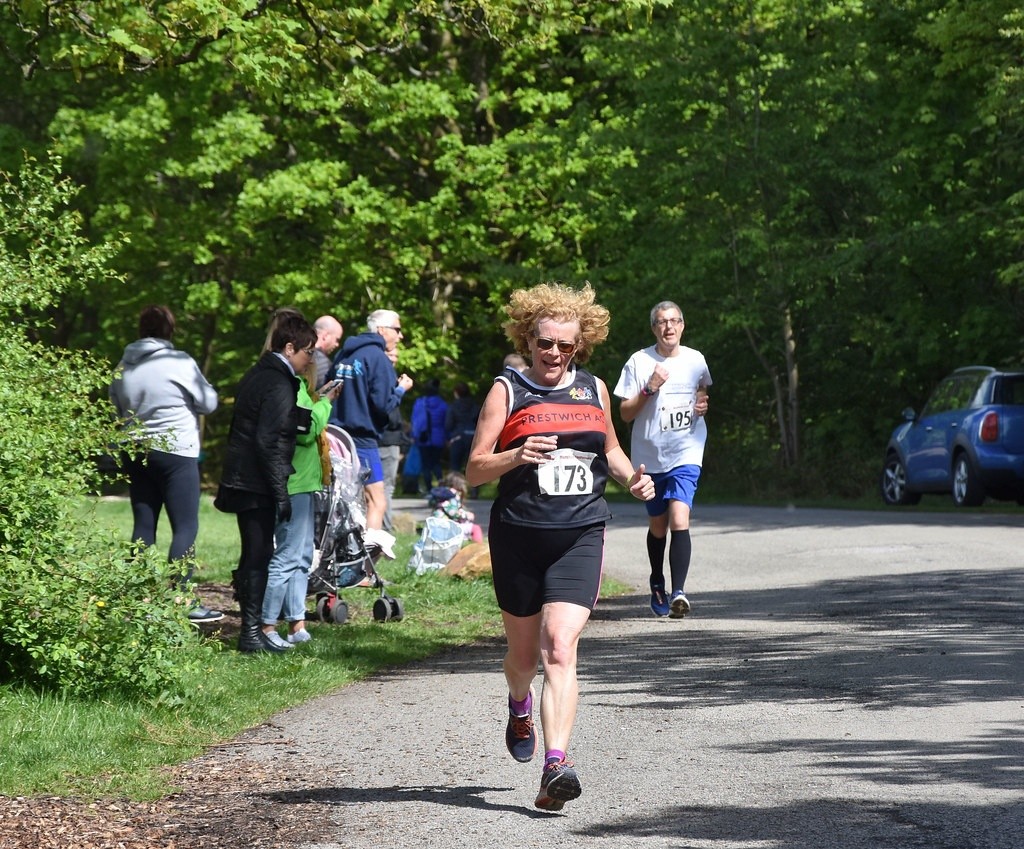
[422,446,443,461]
[410,517,463,575]
[314,392,333,485]
[444,544,491,581]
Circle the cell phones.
[319,379,344,396]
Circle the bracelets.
[642,386,655,397]
[625,475,633,489]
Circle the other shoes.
[372,574,390,586]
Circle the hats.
[364,530,396,559]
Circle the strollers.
[279,425,405,623]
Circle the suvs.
[877,365,1024,509]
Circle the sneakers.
[535,755,582,811]
[287,628,311,642]
[668,590,691,619]
[187,599,224,622]
[650,584,671,618]
[266,631,295,650]
[505,685,538,763]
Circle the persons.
[445,382,479,500]
[108,304,225,623]
[261,357,342,650]
[465,284,655,811]
[376,347,408,532]
[613,301,713,618]
[313,315,343,392]
[434,471,481,543]
[213,311,318,654]
[324,309,414,587]
[410,377,449,492]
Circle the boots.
[232,570,289,653]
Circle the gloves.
[279,500,291,525]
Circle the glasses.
[301,348,315,357]
[381,327,401,334]
[655,317,683,326]
[534,331,579,354]
